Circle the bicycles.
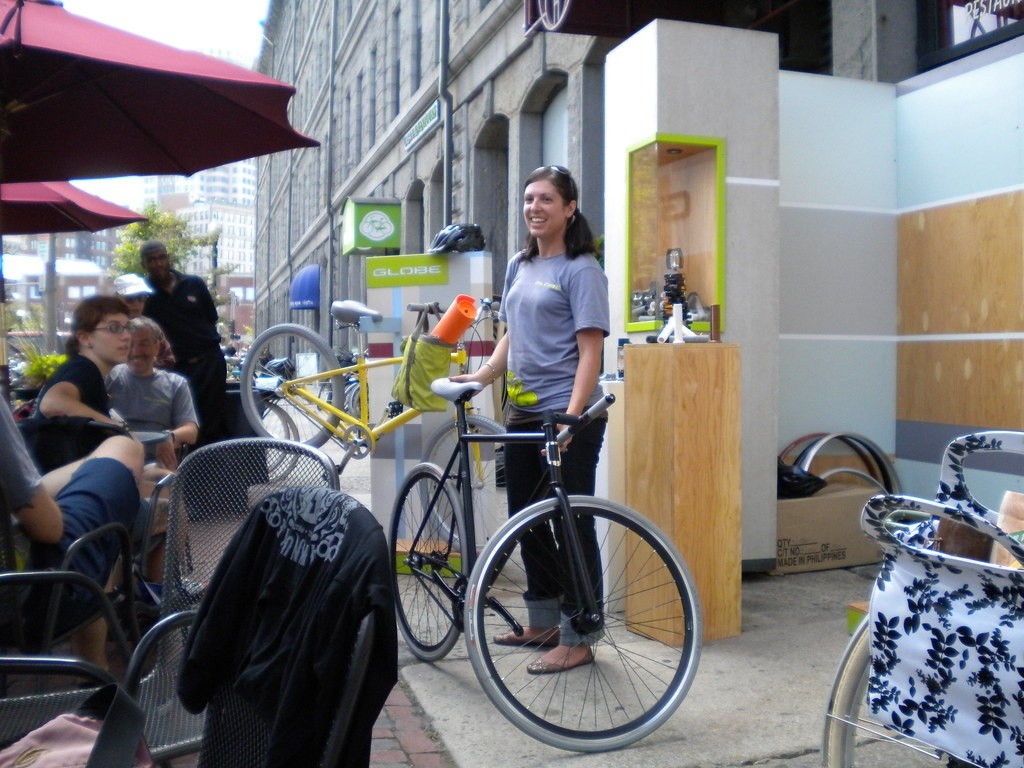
[389,375,702,748]
[255,400,301,483]
[822,610,1023,768]
[317,346,370,420]
[236,298,509,553]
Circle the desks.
[120,431,171,634]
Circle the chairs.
[0,400,398,768]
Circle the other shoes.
[493,629,560,647]
[527,647,594,674]
[156,672,179,716]
[172,576,209,605]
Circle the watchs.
[122,422,130,432]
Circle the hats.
[113,274,153,297]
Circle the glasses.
[93,324,136,334]
[125,296,146,304]
[532,165,574,198]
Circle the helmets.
[427,224,485,254]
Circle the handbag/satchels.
[0,683,154,768]
[860,430,1024,768]
[392,302,453,412]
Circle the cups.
[989,491,1024,569]
[933,515,994,563]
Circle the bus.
[5,330,70,359]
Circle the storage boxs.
[770,481,890,579]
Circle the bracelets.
[481,363,496,384]
[161,430,175,443]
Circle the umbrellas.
[0,0,320,574]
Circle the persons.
[140,241,227,520]
[36,273,200,696]
[256,347,275,377]
[449,166,610,673]
[0,393,172,715]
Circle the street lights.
[208,219,224,292]
